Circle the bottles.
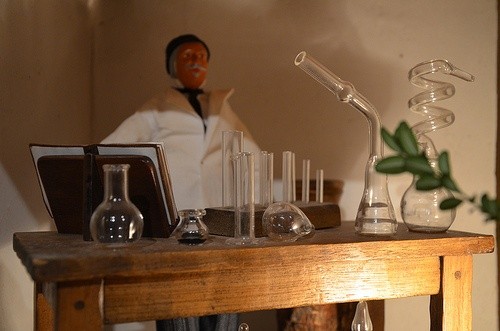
[89,164,144,246]
[174,209,209,245]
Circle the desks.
[12,217,495,331]
